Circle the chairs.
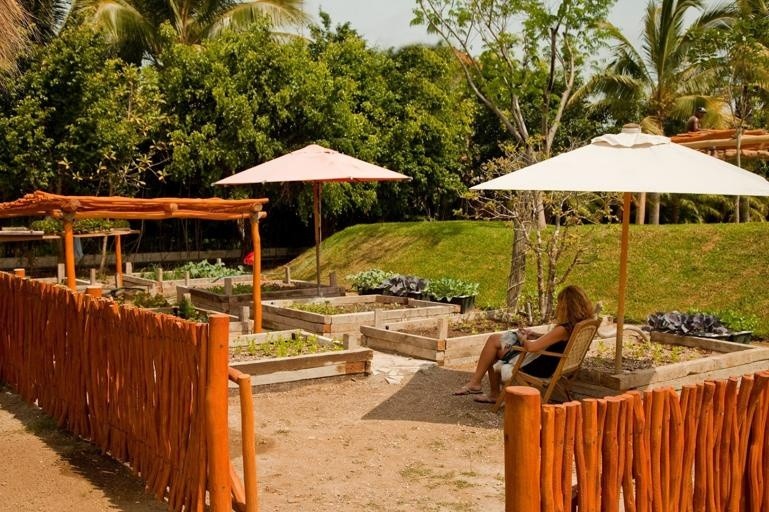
[492,317,605,416]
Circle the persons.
[454,285,595,402]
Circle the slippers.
[474,395,497,403]
[452,386,482,394]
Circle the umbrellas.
[469,122,769,374]
[212,144,409,284]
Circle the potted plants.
[644,306,753,344]
[350,268,481,315]
